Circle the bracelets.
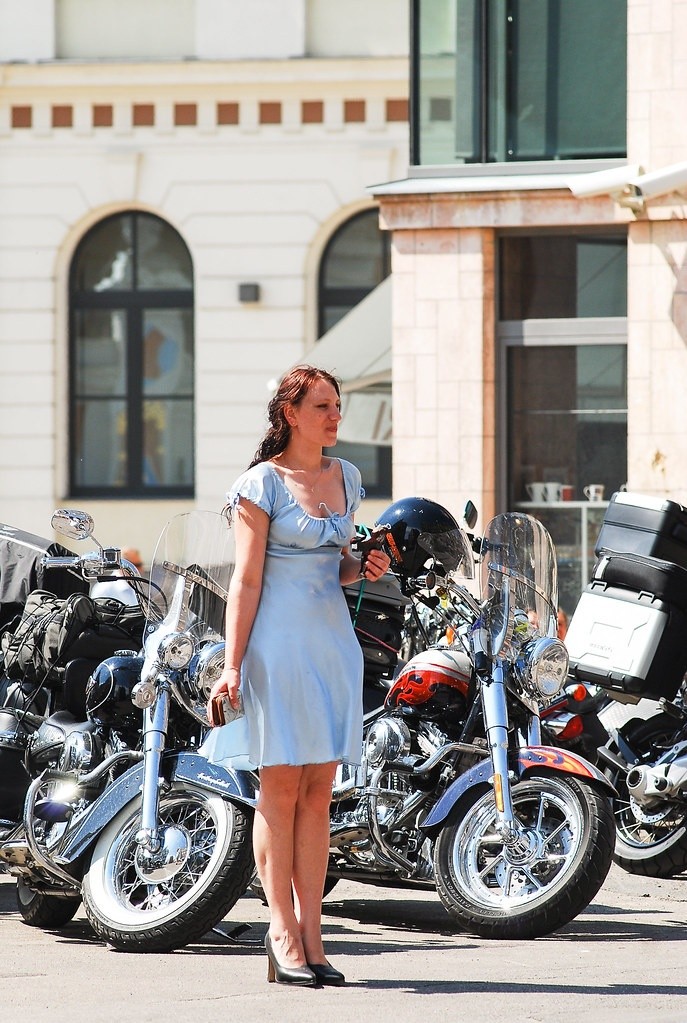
[222,667,238,672]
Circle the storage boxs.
[563,580,687,703]
[340,571,405,642]
[593,489,687,558]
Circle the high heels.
[307,957,345,986]
[266,932,318,986]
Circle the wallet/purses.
[212,690,244,726]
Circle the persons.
[206,368,391,984]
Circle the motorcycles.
[0,496,687,954]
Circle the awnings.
[270,277,391,445]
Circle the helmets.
[375,496,464,597]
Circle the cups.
[583,484,605,501]
[559,484,578,501]
[525,481,544,502]
[542,482,563,502]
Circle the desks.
[514,500,609,592]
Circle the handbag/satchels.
[2,590,150,687]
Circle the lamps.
[565,161,687,215]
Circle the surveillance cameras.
[565,166,641,198]
[628,163,687,201]
